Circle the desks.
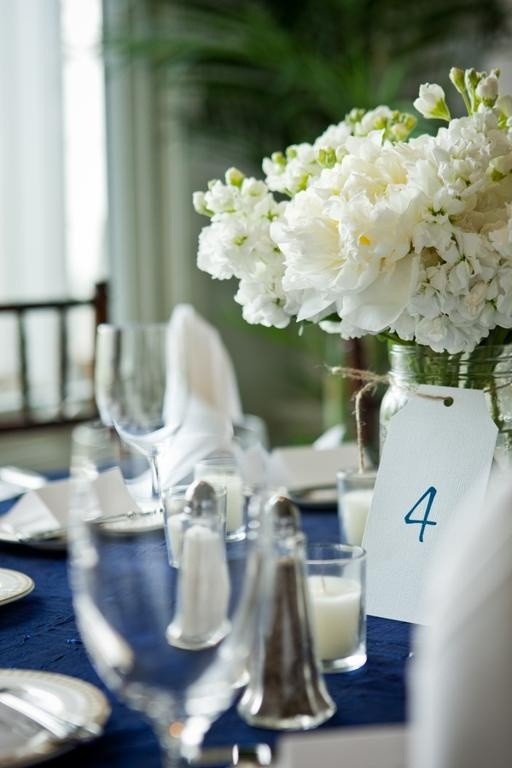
[1,465,426,767]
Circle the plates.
[0,664,112,767]
[0,566,37,608]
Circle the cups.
[295,540,370,676]
[334,470,378,542]
[64,418,284,768]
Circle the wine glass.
[91,318,197,521]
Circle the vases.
[379,343,511,464]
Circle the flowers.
[192,67,512,465]
